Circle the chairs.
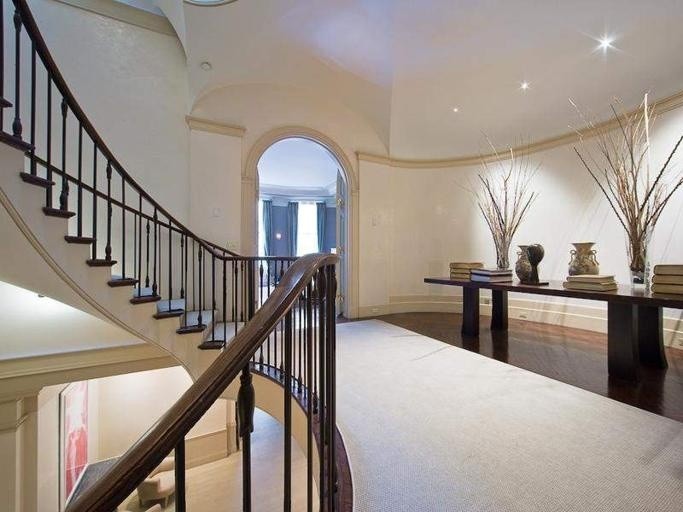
[136,456,174,507]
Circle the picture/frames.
[57,380,90,511]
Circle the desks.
[424,275,682,371]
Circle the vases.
[514,245,534,282]
[567,241,599,276]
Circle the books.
[651,264,683,295]
[562,275,618,291]
[450,263,514,283]
